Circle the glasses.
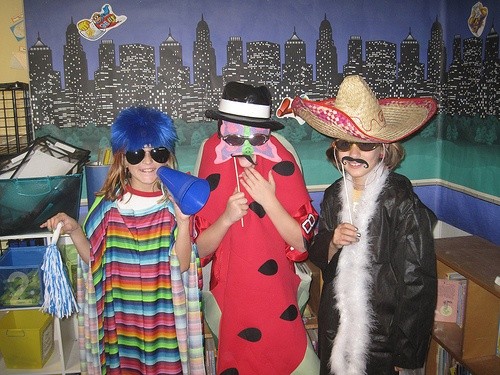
[124,146,170,165]
[334,139,379,152]
[219,129,271,146]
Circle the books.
[434,273,473,375]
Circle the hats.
[206,82,285,131]
[293,75,437,143]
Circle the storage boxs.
[0,246,47,308]
[0,310,54,369]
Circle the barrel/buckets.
[85,160,111,211]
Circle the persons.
[40,104,207,375]
[290,74,438,375]
[192,81,321,375]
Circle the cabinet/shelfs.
[0,233,81,375]
[424,235,500,375]
